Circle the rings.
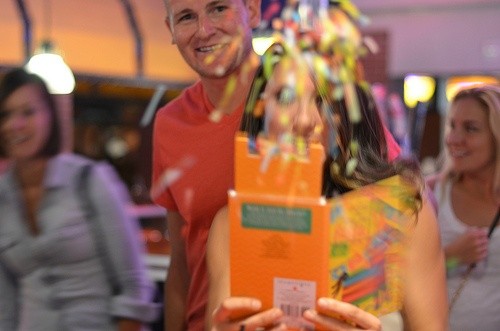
[239,319,246,331]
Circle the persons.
[151,1,403,331]
[421,84,500,331]
[0,68,162,331]
[206,46,446,331]
